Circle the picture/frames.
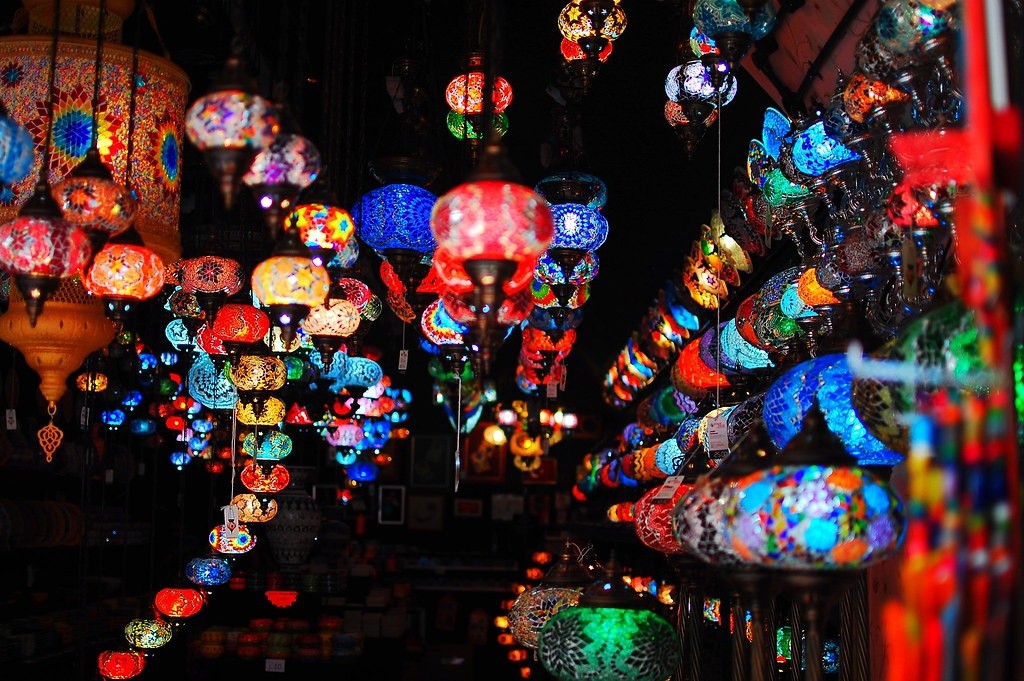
[377,485,406,526]
[409,434,452,491]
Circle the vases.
[268,467,322,567]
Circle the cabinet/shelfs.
[405,564,521,596]
